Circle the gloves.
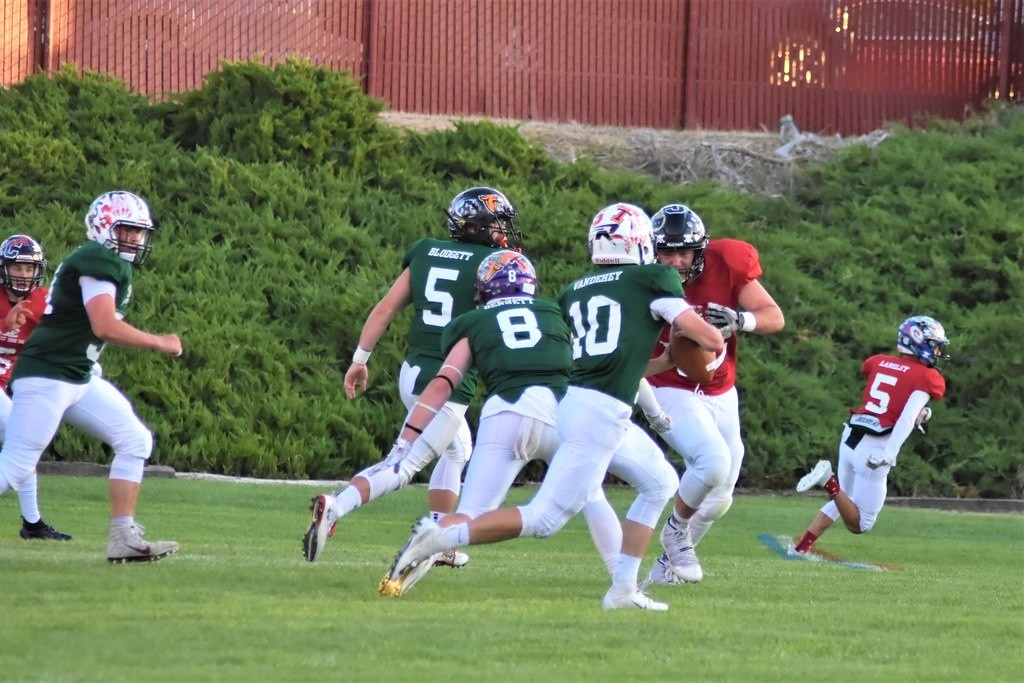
[367,437,413,477]
[705,303,745,335]
[646,409,672,434]
[865,455,888,470]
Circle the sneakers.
[389,516,455,583]
[649,517,703,585]
[378,544,442,598]
[602,588,669,611]
[301,491,337,563]
[787,545,807,556]
[20,515,72,542]
[107,516,179,564]
[796,460,835,493]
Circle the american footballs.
[669,321,717,385]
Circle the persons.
[369,205,784,611]
[787,314,947,563]
[0,234,73,543]
[302,186,513,568]
[0,189,183,563]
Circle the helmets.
[589,203,654,264]
[476,250,537,306]
[85,191,155,254]
[0,235,47,285]
[897,316,950,368]
[448,187,517,247]
[651,204,706,270]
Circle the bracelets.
[740,311,756,332]
[353,345,371,364]
[404,423,423,435]
